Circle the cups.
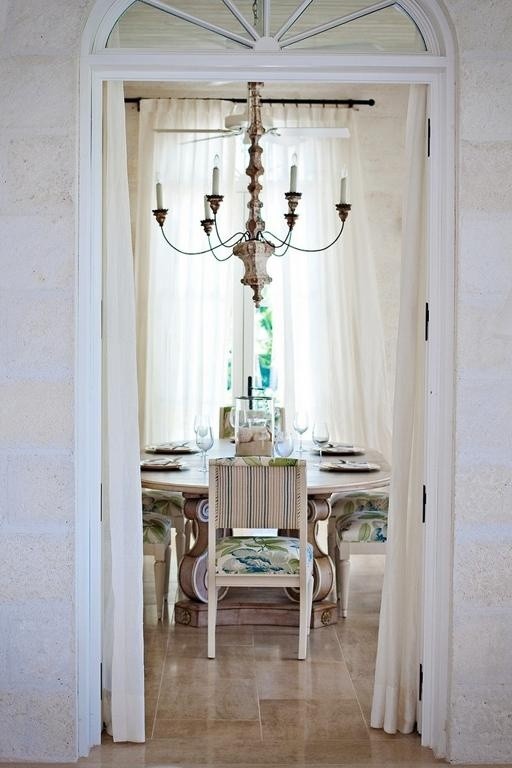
[274,430,295,458]
[229,394,276,456]
[193,414,211,434]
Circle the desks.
[140,435,389,629]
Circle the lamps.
[152,82,352,309]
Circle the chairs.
[205,455,315,660]
[144,488,194,624]
[327,490,389,619]
[217,406,286,437]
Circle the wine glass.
[196,426,214,472]
[312,421,330,465]
[292,414,311,453]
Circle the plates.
[140,458,184,470]
[144,444,193,453]
[318,461,380,473]
[313,445,366,454]
